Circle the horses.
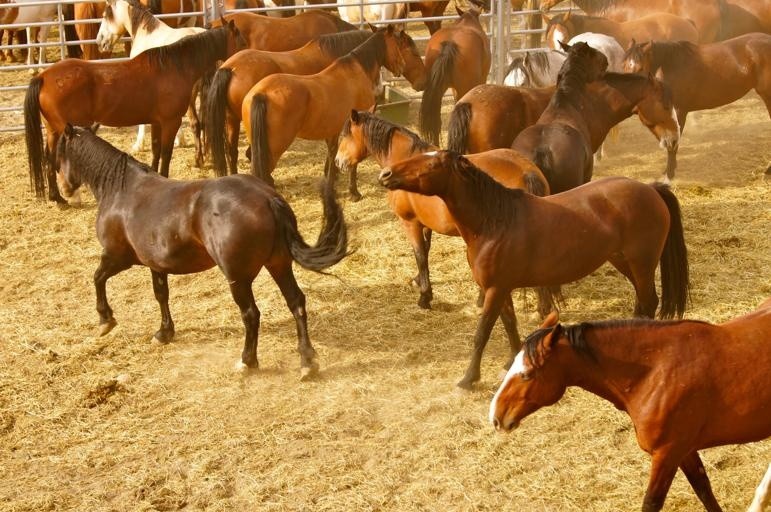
[336,106,568,325]
[0,0,771,210]
[375,146,690,401]
[55,124,352,384]
[487,301,771,512]
